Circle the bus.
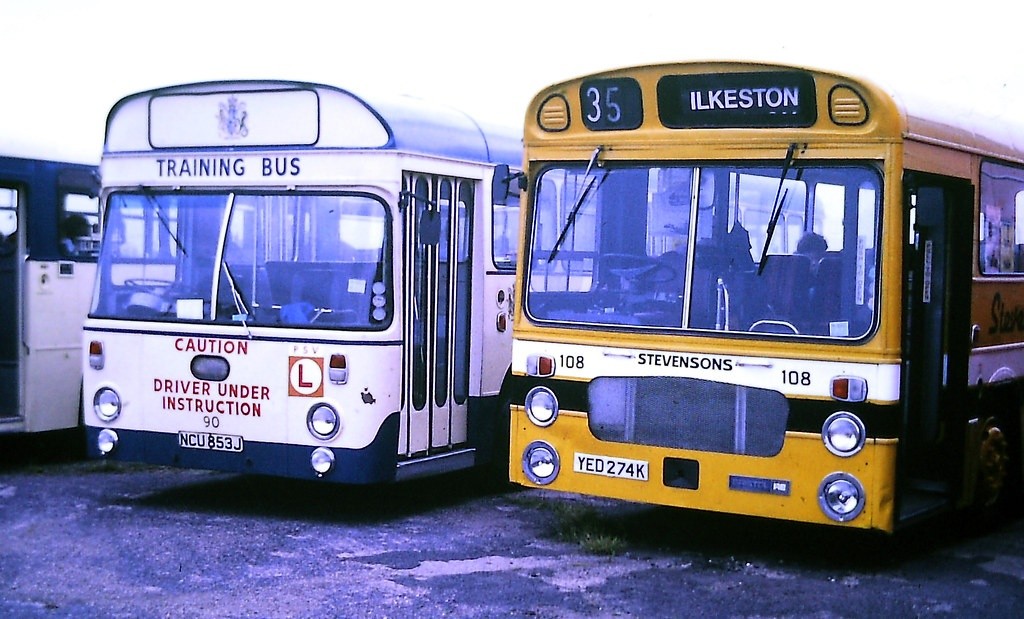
[509,57,1023,544]
[0,136,387,440]
[81,79,823,495]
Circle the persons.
[790,232,829,307]
